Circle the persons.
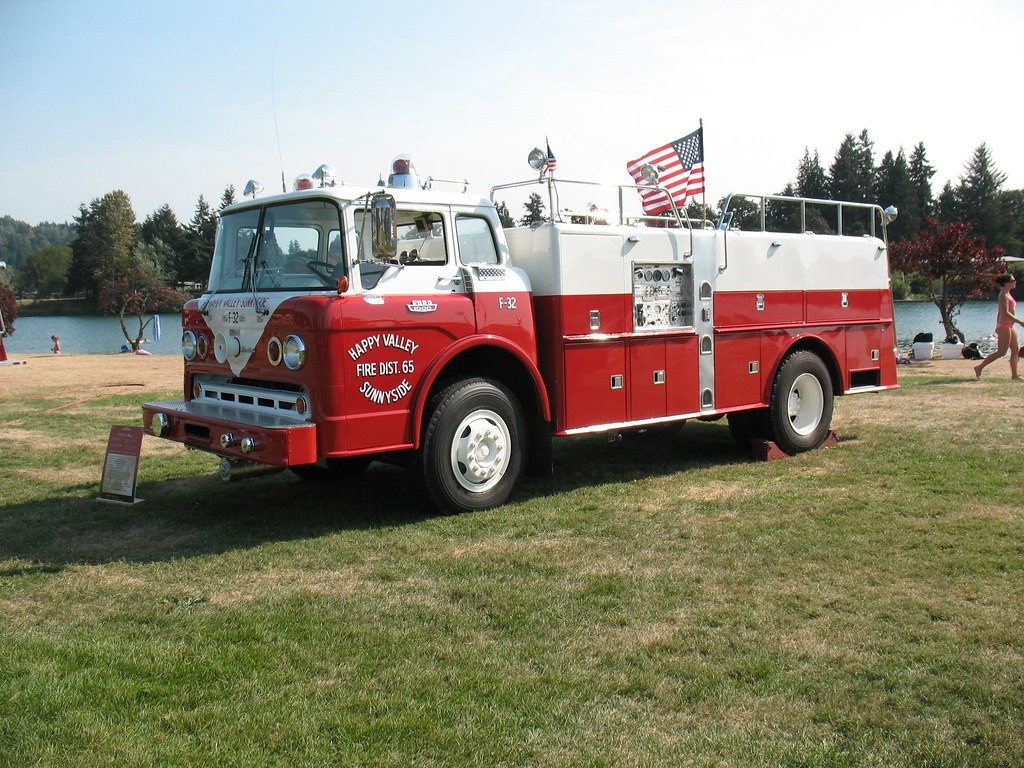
[974,273,1024,382]
[50,335,61,353]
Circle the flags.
[544,144,557,173]
[627,126,705,217]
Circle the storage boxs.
[912,342,934,360]
[940,342,964,360]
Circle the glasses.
[1010,280,1016,282]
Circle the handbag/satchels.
[914,333,932,342]
[962,343,985,359]
[945,336,958,344]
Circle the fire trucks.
[141,137,901,518]
